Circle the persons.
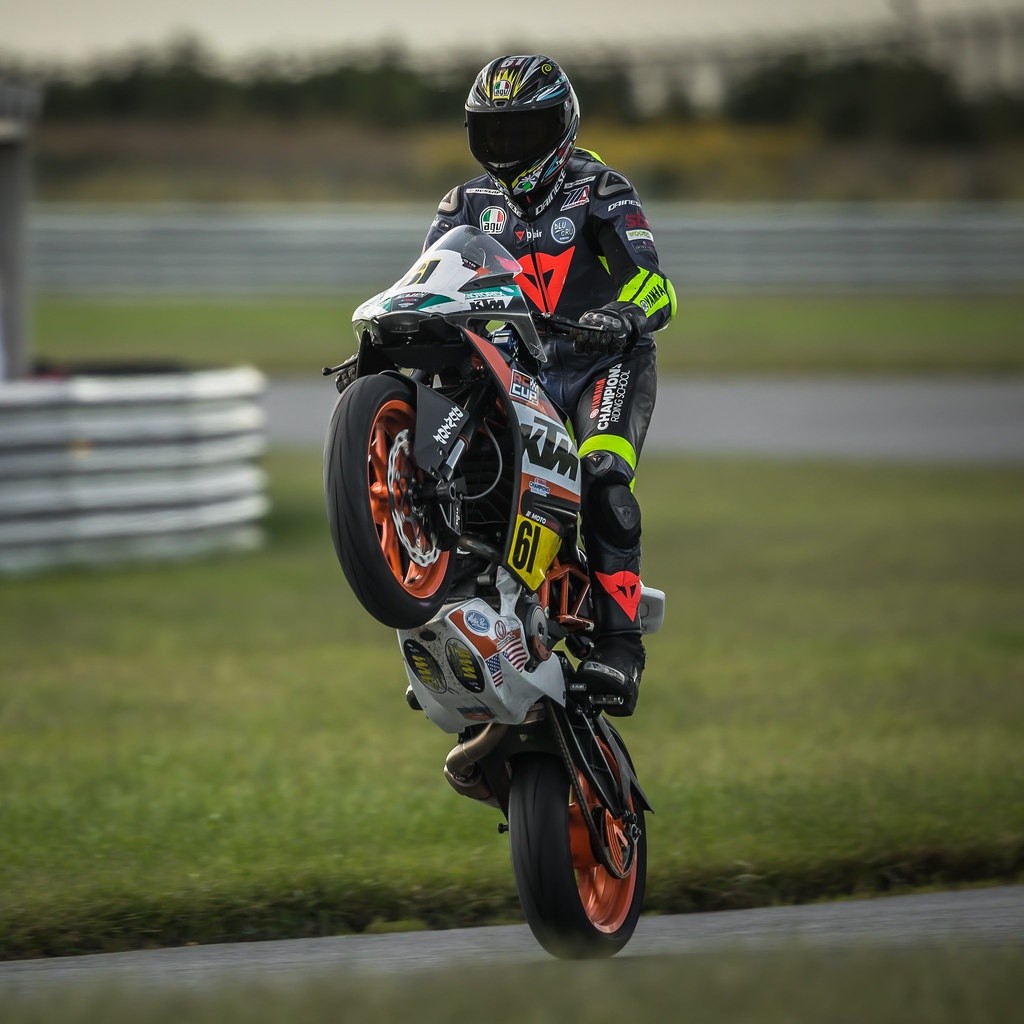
[335,53,680,718]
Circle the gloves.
[571,301,647,357]
[335,351,359,394]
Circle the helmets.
[463,53,582,221]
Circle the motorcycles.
[322,225,665,960]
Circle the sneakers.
[405,683,424,714]
[578,642,646,717]
